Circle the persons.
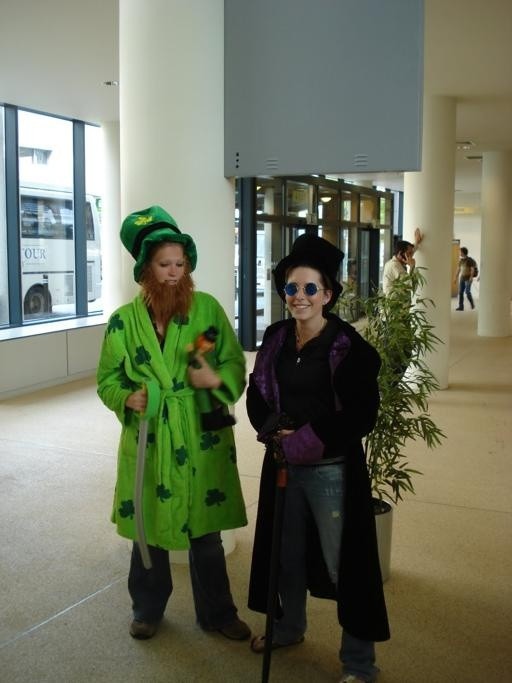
[182,326,236,434]
[382,226,424,392]
[451,246,479,310]
[243,234,391,683]
[95,207,252,642]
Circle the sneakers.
[457,307,463,310]
[216,617,252,640]
[129,617,157,640]
[339,666,381,683]
[250,632,305,653]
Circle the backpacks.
[460,258,478,278]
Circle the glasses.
[282,282,325,296]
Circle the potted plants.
[329,265,448,586]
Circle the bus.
[17,184,105,322]
[234,207,267,292]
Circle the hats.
[120,205,198,283]
[273,233,345,312]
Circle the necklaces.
[295,317,323,346]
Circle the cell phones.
[400,251,409,261]
